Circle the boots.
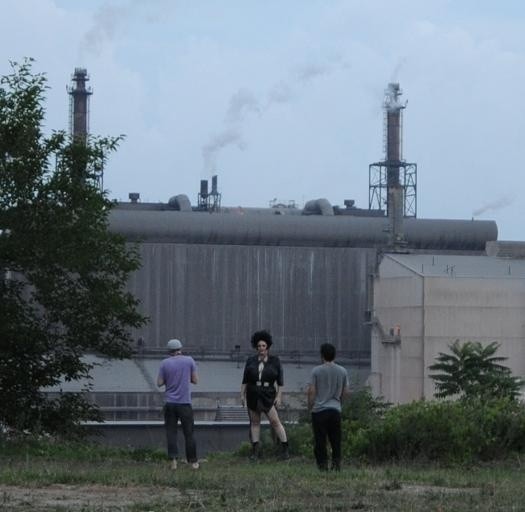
[251,441,260,460]
[281,442,290,459]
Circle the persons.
[155,338,202,472]
[240,328,291,462]
[304,342,351,473]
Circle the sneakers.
[170,459,199,470]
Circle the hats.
[166,339,182,350]
